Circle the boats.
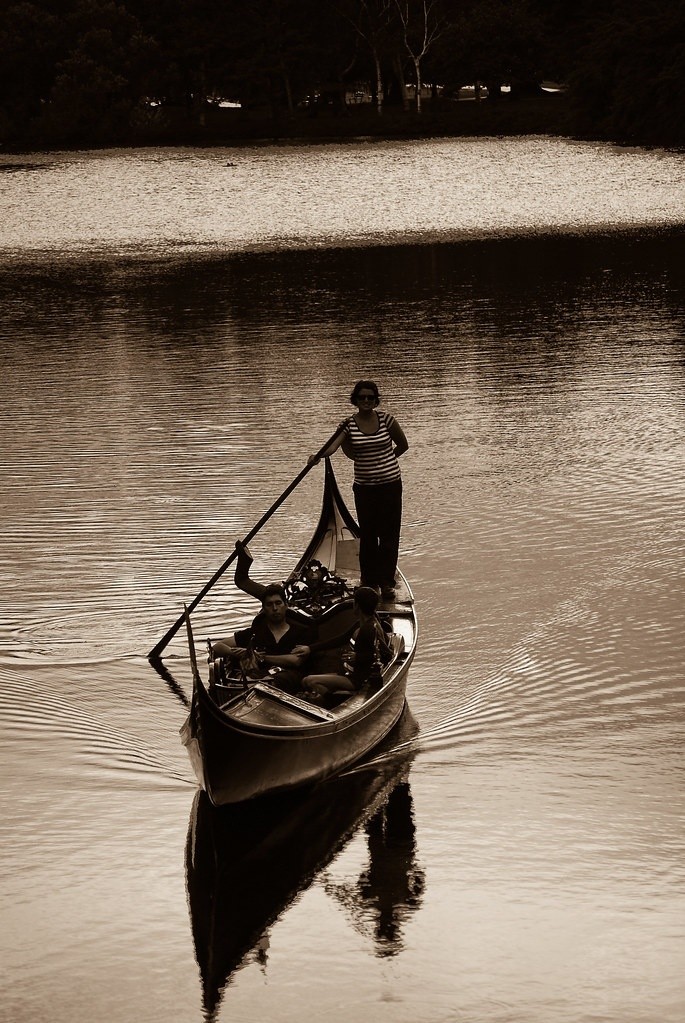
[182,698,425,1023]
[180,458,419,807]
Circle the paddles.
[148,419,351,657]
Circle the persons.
[308,381,409,600]
[212,583,304,696]
[291,587,382,695]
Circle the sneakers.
[380,584,396,599]
[360,581,379,592]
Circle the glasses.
[357,394,375,401]
